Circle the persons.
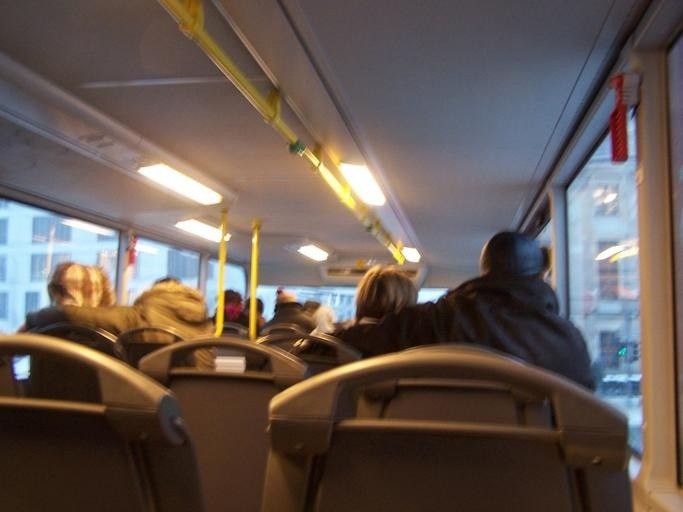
[313,305,336,332]
[16,261,128,334]
[289,230,596,425]
[304,300,320,314]
[133,275,215,340]
[244,297,265,326]
[84,265,149,330]
[211,289,260,334]
[260,288,318,334]
[353,263,418,319]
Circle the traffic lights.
[618,342,640,363]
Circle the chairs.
[0,290,634,512]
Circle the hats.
[480,231,544,277]
